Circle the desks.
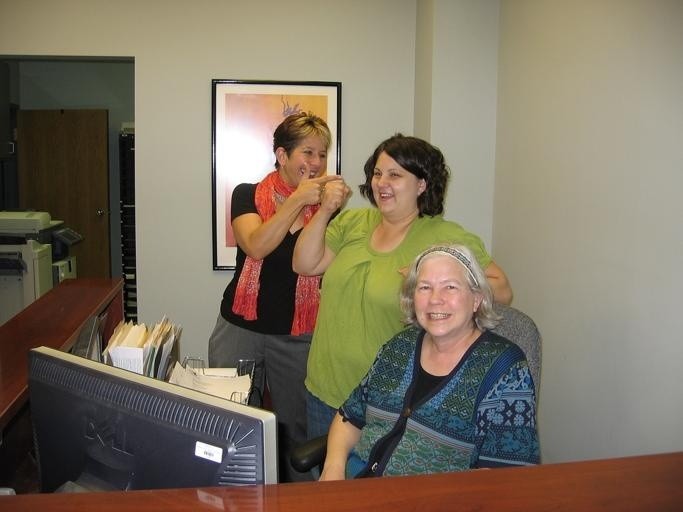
[0,276,682,510]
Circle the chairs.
[289,300,542,478]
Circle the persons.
[318,242,542,481]
[291,133,512,482]
[208,110,343,482]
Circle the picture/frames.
[209,77,341,271]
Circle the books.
[102,312,252,404]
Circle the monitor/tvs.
[25,342,279,493]
